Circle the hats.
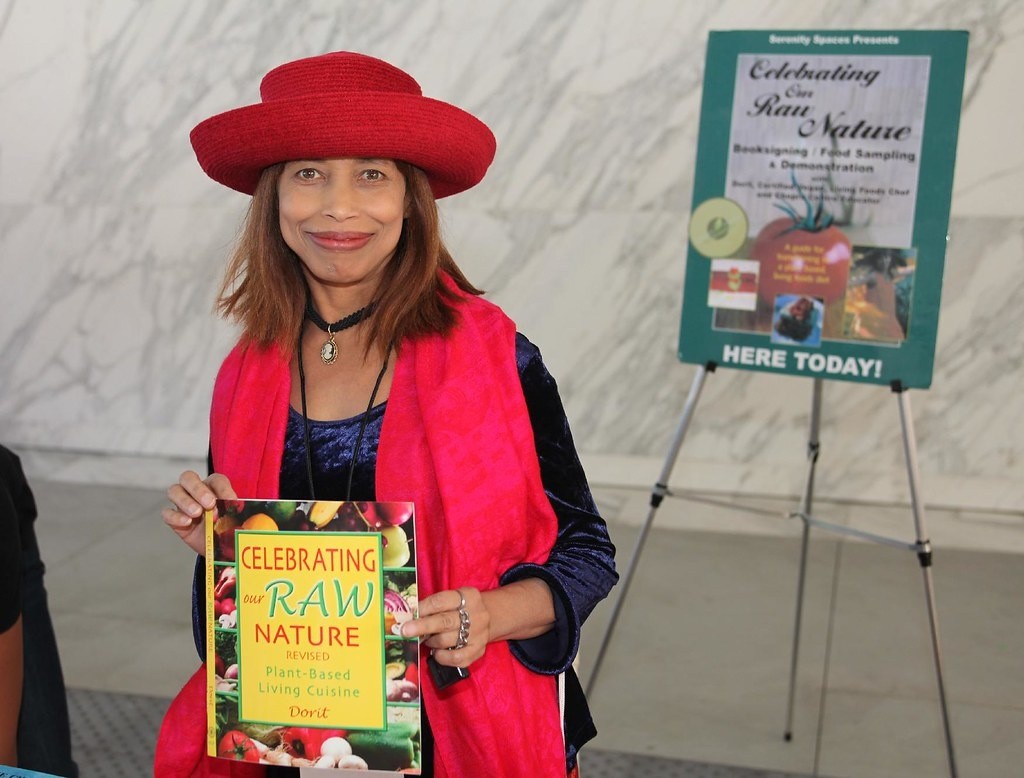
[188,50,497,201]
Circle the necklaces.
[297,288,402,500]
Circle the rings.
[453,585,467,609]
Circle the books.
[204,493,426,778]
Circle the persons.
[0,446,83,778]
[134,50,620,775]
